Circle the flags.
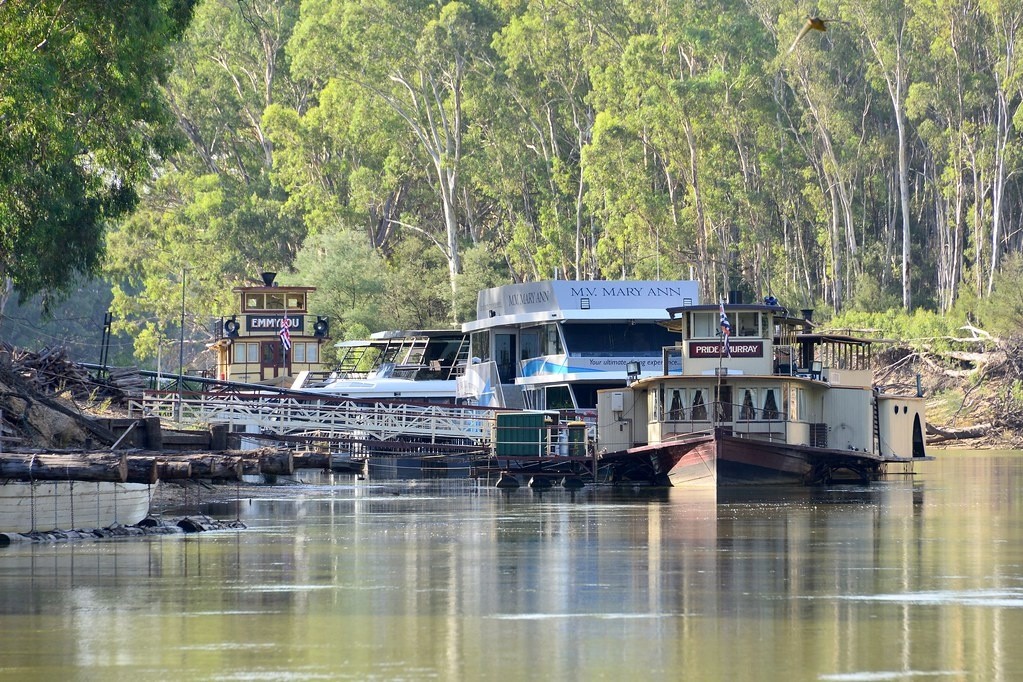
[280,311,291,350]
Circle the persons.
[264,355,267,362]
[280,354,283,362]
[720,297,732,359]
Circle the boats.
[597,294,935,486]
[206,272,700,456]
